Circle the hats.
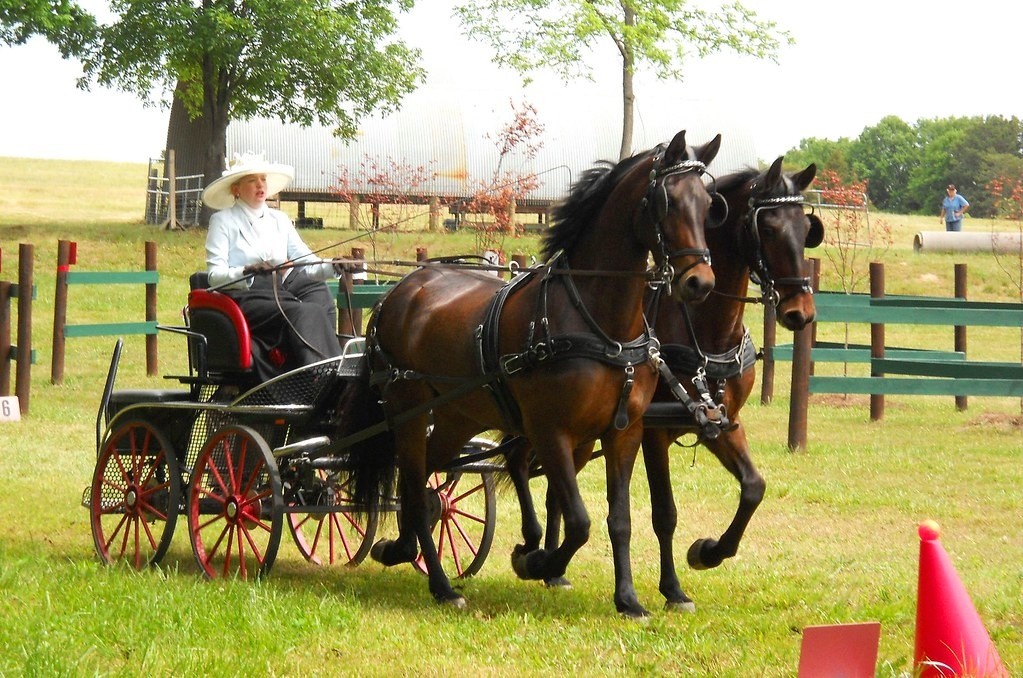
[947,184,956,191]
[201,155,295,210]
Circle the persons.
[201,154,365,405]
[939,185,970,230]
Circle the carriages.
[81,128,825,621]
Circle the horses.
[338,130,720,625]
[480,156,817,611]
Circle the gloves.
[332,258,364,274]
[244,259,285,277]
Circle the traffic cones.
[916,519,1012,678]
